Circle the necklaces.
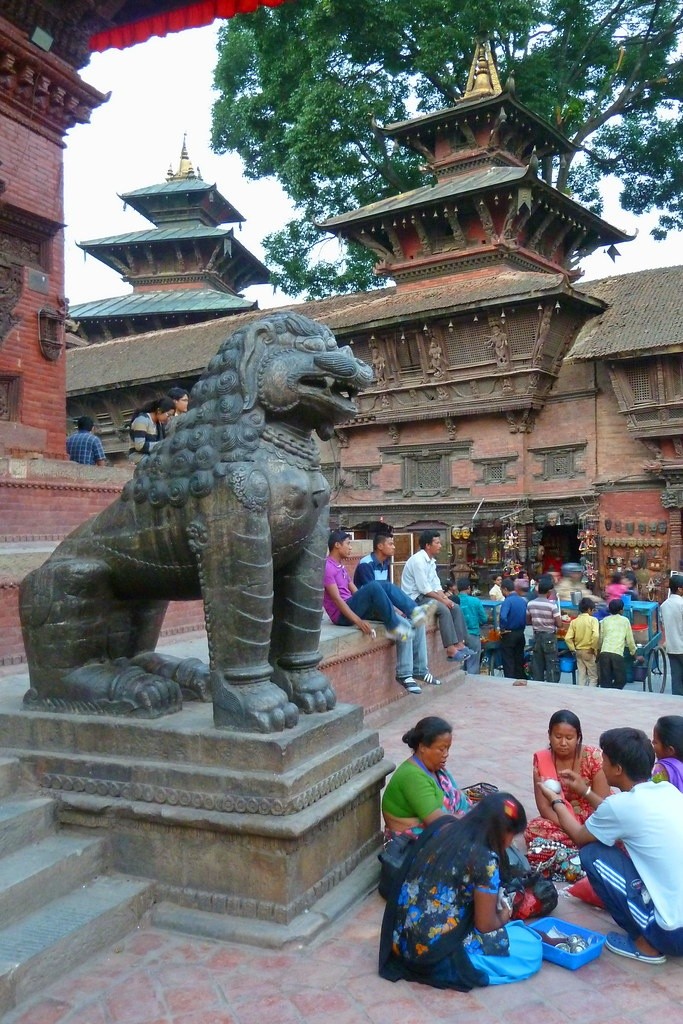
[627,781,648,792]
[554,749,576,802]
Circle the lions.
[20,313,376,732]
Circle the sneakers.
[411,599,437,629]
[385,625,415,642]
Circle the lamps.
[28,25,54,52]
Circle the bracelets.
[443,596,447,599]
[552,798,566,812]
[585,787,591,797]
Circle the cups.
[621,594,631,606]
[570,591,582,605]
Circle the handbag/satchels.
[377,834,416,899]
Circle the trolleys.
[596,594,670,694]
[465,599,533,677]
[525,599,598,686]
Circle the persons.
[168,387,189,415]
[488,563,639,601]
[402,532,477,661]
[524,709,616,848]
[660,574,683,696]
[537,727,683,964]
[382,716,472,848]
[652,715,683,793]
[354,532,442,694]
[499,575,644,690]
[323,529,437,642]
[125,398,177,465]
[66,415,106,467]
[445,577,488,674]
[378,791,543,993]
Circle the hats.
[457,578,475,590]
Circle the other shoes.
[413,673,440,685]
[396,676,421,693]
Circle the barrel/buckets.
[632,659,648,681]
[631,624,650,646]
[558,650,577,672]
[547,571,559,583]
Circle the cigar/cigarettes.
[452,605,454,606]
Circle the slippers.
[458,647,478,655]
[605,931,666,964]
[447,651,471,661]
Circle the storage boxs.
[527,916,607,971]
